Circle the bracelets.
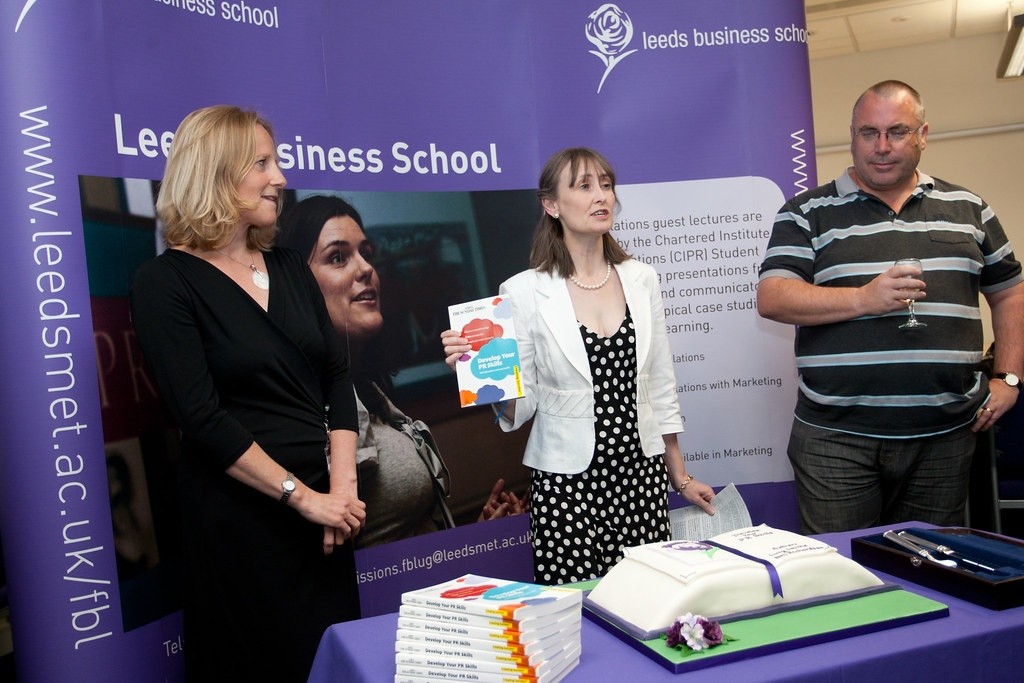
[674,474,694,496]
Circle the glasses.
[854,124,923,142]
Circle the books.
[444,294,525,409]
[393,571,584,683]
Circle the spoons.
[883,529,958,568]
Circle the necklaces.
[213,246,272,291]
[569,259,612,290]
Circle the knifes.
[897,531,1013,575]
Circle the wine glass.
[892,259,928,330]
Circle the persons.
[129,102,367,683]
[275,195,535,541]
[752,80,1024,535]
[440,147,715,585]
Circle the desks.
[323,518,1024,683]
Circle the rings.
[982,407,993,414]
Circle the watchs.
[988,372,1024,396]
[278,469,296,506]
